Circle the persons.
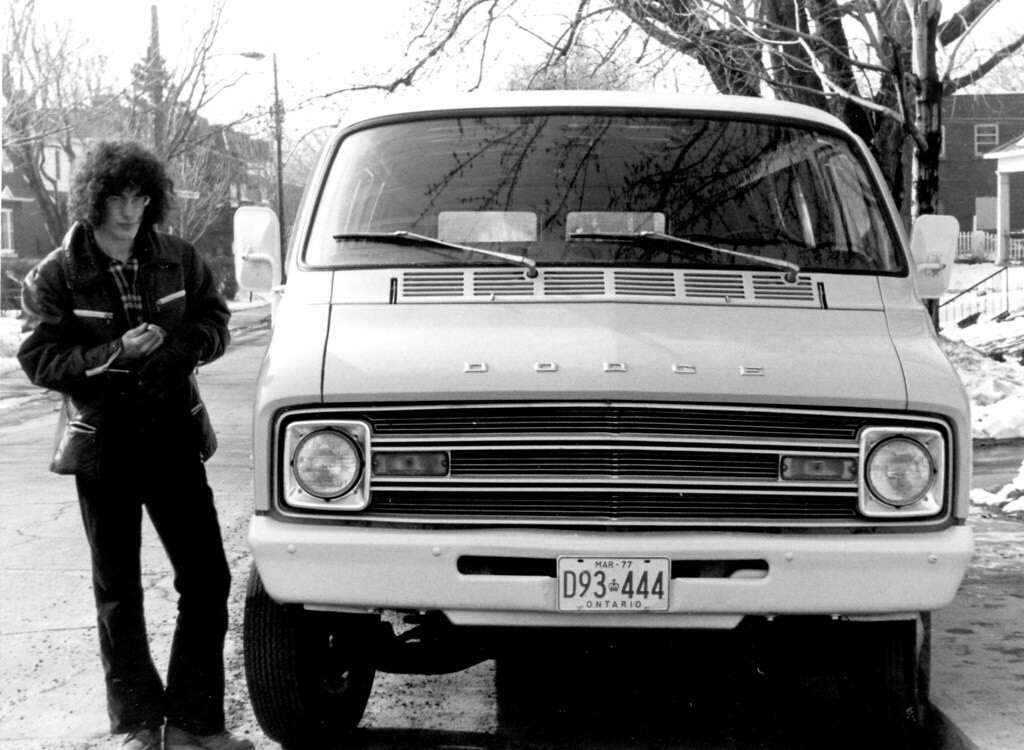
[17,139,255,750]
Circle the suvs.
[236,89,979,743]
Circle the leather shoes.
[164,725,255,750]
[117,721,162,750]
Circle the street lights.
[238,51,288,287]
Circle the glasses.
[105,195,151,209]
